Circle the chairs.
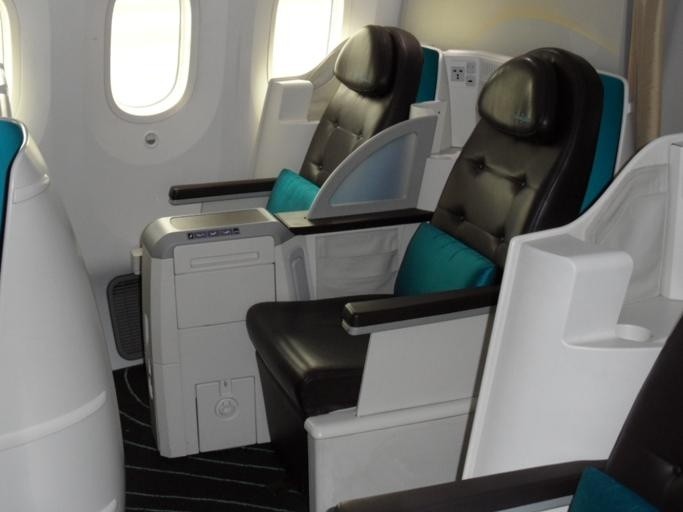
[110,26,444,450]
[248,41,683,512]
